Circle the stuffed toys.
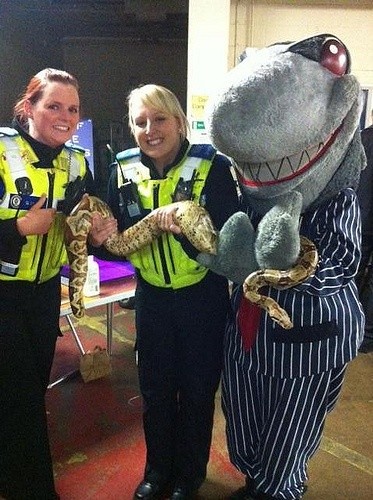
[208,33,366,500]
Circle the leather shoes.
[134,480,161,500]
[169,484,192,500]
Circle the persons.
[86,85,241,500]
[0,68,95,500]
[355,109,373,354]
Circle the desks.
[45,279,137,389]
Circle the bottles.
[83,255,99,296]
